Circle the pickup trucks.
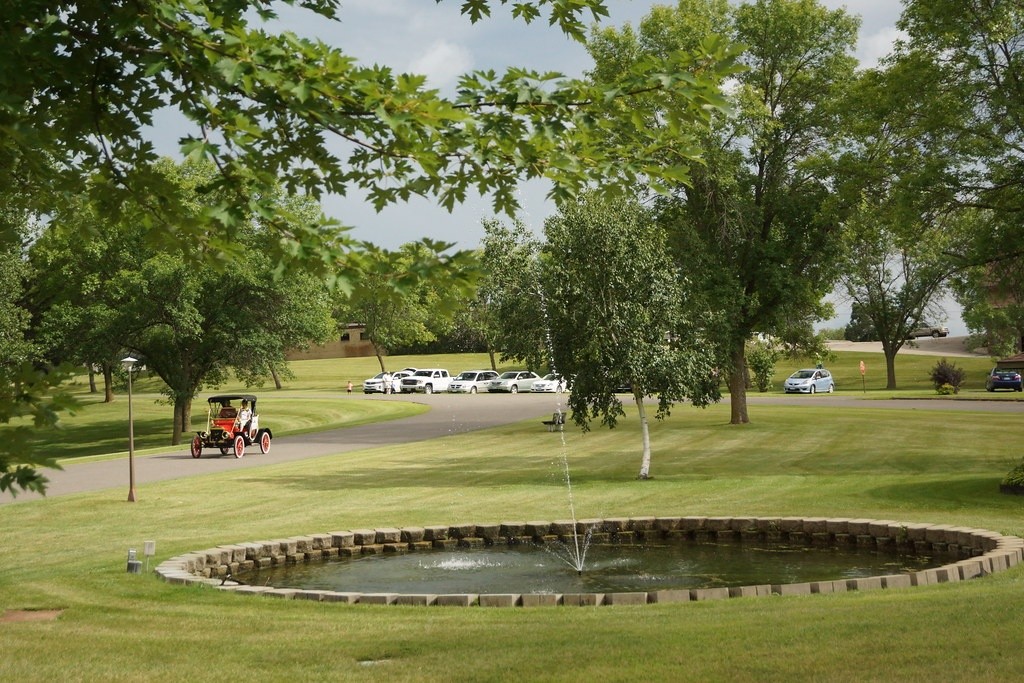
[398,367,463,393]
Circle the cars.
[363,372,410,395]
[191,391,273,459]
[613,372,657,392]
[447,369,501,394]
[529,372,578,396]
[487,370,541,394]
[986,369,1023,394]
[783,368,836,395]
[401,367,417,375]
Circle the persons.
[347,381,353,395]
[383,371,401,394]
[219,399,237,418]
[814,352,823,369]
[234,399,252,432]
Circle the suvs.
[905,320,950,339]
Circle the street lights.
[121,356,139,500]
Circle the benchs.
[541,412,567,432]
[211,417,239,431]
